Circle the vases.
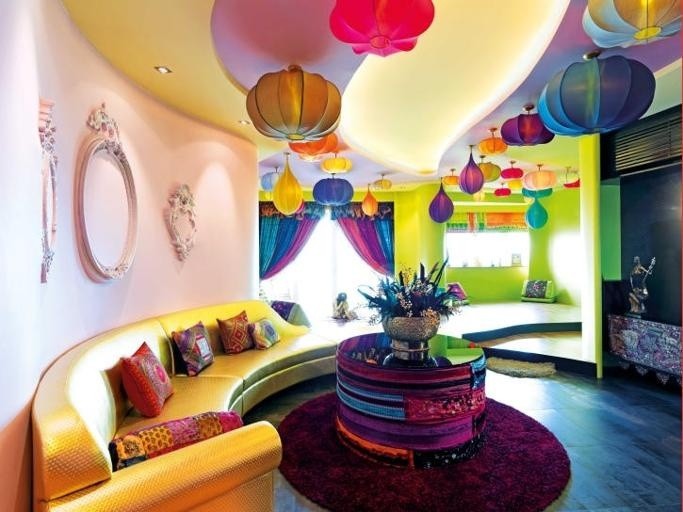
[379,314,443,350]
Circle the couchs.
[28,297,337,512]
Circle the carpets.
[280,390,572,511]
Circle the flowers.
[353,257,466,317]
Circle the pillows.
[216,309,282,354]
[109,410,242,471]
[170,319,215,380]
[121,340,174,416]
